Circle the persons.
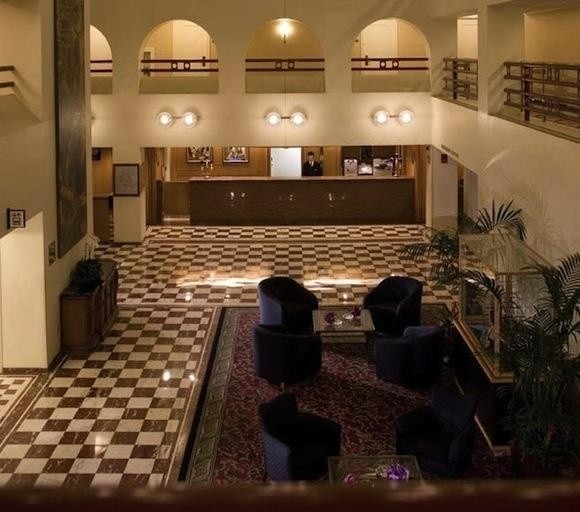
[302,152,323,175]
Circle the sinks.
[375,163,392,170]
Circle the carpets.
[179,304,577,483]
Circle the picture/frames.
[113,164,139,196]
[6,208,26,229]
[222,146,249,162]
[186,147,213,163]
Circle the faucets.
[383,156,394,165]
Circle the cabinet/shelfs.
[60,258,119,355]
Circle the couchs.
[395,396,476,479]
[363,276,422,326]
[258,391,341,482]
[254,325,321,384]
[258,277,318,330]
[376,326,445,390]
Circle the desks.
[313,308,376,356]
[328,455,426,488]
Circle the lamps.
[278,20,293,44]
[290,112,306,129]
[157,112,173,128]
[399,110,415,127]
[373,111,389,127]
[265,112,282,128]
[181,112,197,128]
